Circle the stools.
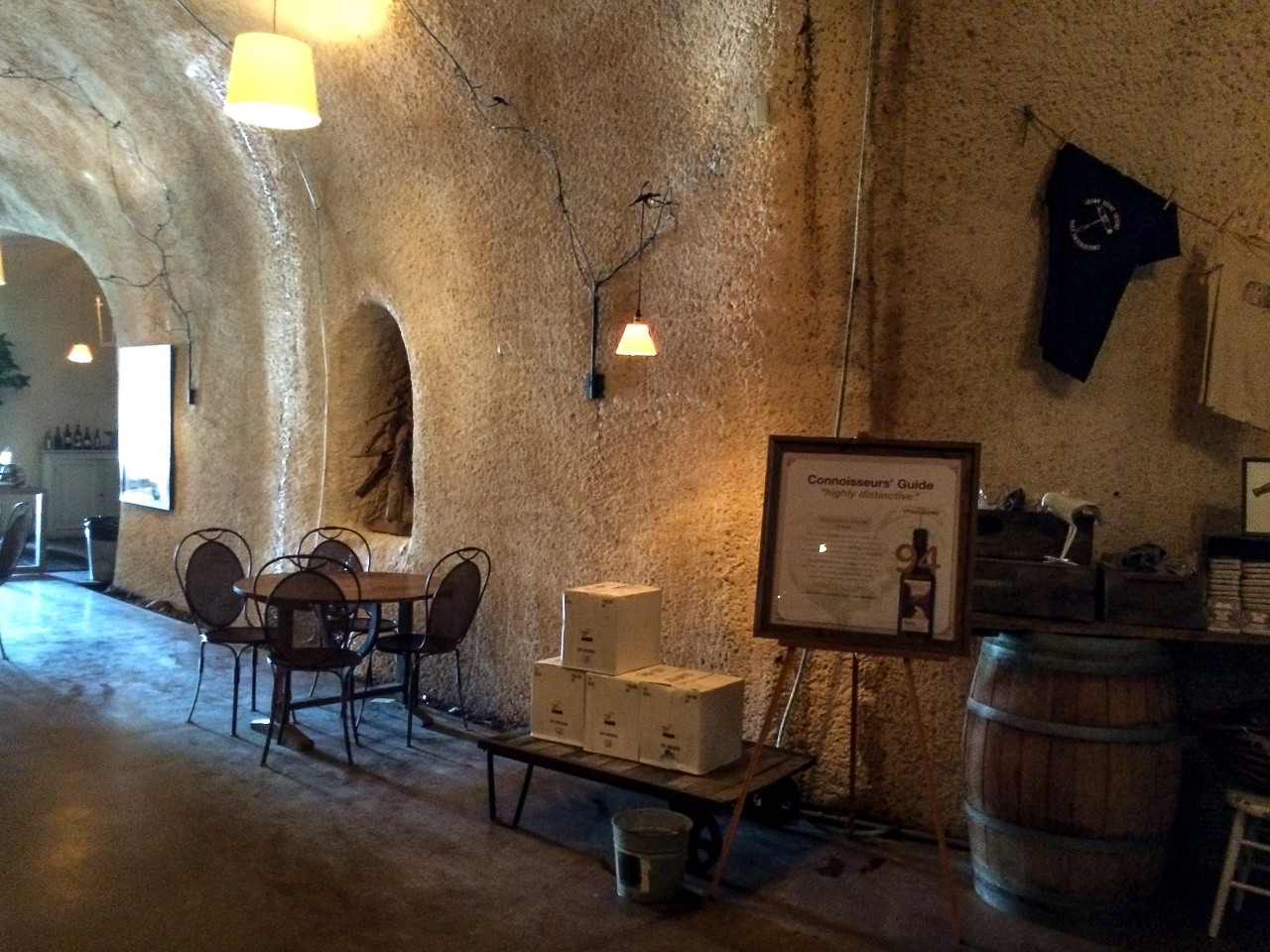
[1208,784,1270,939]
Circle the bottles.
[45,431,52,450]
[54,426,61,449]
[94,429,100,448]
[64,424,72,449]
[84,427,91,449]
[74,424,82,449]
[898,528,935,634]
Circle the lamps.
[222,0,322,131]
[66,341,93,364]
[615,197,659,356]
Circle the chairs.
[256,554,362,768]
[0,501,33,661]
[297,524,417,719]
[353,546,492,749]
[173,528,298,739]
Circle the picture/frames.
[753,436,983,660]
[1241,457,1270,538]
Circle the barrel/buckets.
[610,807,694,903]
[963,633,1185,925]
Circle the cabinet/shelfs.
[40,448,123,541]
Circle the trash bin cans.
[85,516,118,581]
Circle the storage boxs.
[559,580,661,676]
[530,656,588,748]
[638,669,746,776]
[585,665,685,763]
[972,507,1210,633]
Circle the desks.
[0,484,47,574]
[476,727,818,881]
[232,570,446,754]
